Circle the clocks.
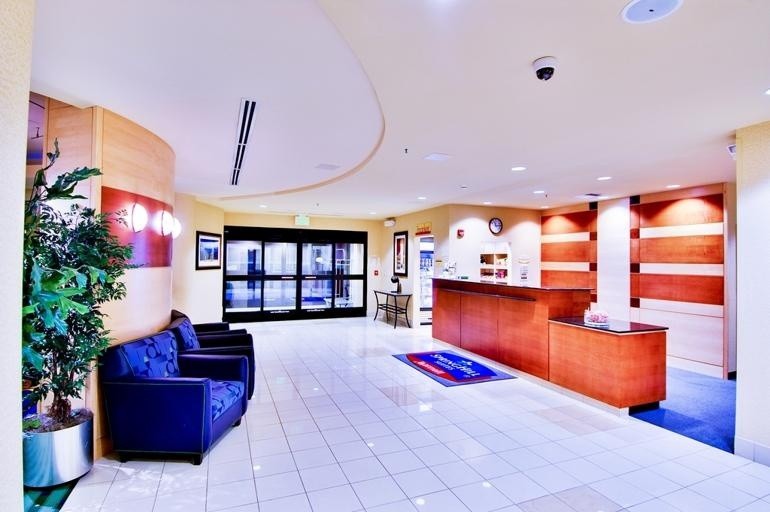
[489,217,503,234]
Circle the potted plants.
[23,138,144,488]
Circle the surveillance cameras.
[534,57,557,82]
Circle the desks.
[373,289,413,329]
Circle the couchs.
[98,309,254,466]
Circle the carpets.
[391,349,518,386]
[630,365,734,454]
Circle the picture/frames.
[195,231,222,270]
[394,231,408,277]
